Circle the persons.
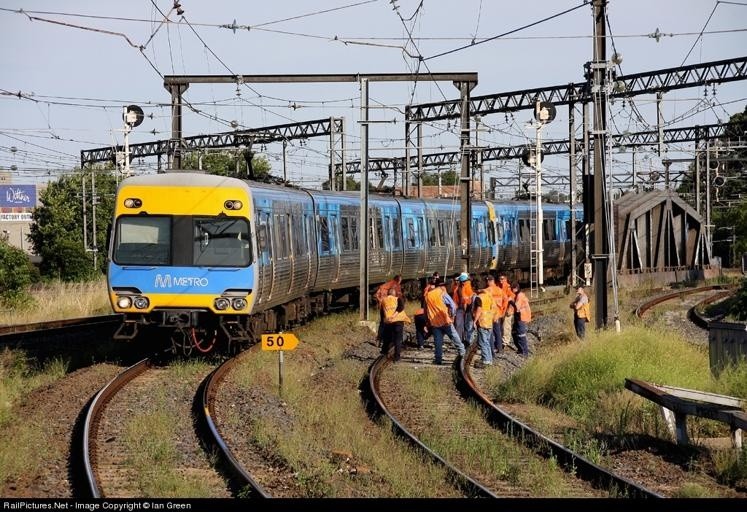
[374,272,531,368]
[570,284,590,340]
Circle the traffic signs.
[260,331,300,352]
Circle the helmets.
[458,275,468,282]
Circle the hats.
[485,275,494,280]
[508,282,520,288]
[573,284,583,289]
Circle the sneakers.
[474,359,490,368]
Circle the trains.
[107,169,585,361]
